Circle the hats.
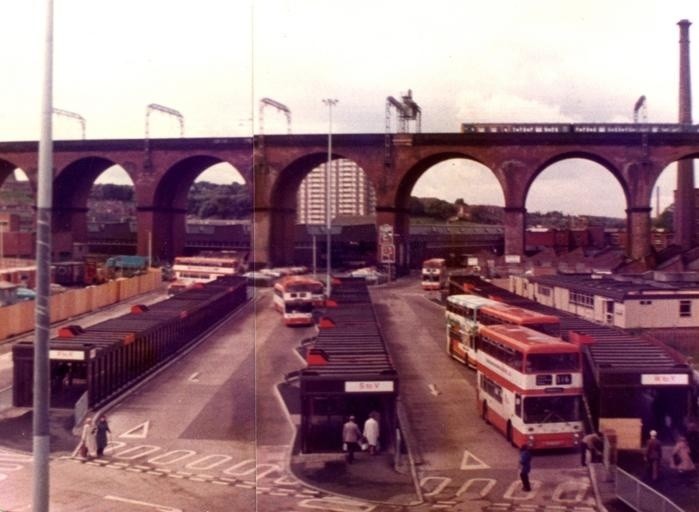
[649,429,658,438]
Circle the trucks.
[105,255,148,277]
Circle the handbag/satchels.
[360,436,369,452]
[343,442,348,451]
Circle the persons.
[645,430,661,480]
[96,416,111,457]
[518,444,531,492]
[79,416,99,464]
[341,415,363,466]
[574,430,586,466]
[667,436,695,473]
[583,433,603,465]
[362,410,381,455]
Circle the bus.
[168,256,240,294]
[445,294,584,451]
[422,258,447,291]
[275,275,325,326]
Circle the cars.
[245,265,307,286]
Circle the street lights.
[322,97,341,295]
